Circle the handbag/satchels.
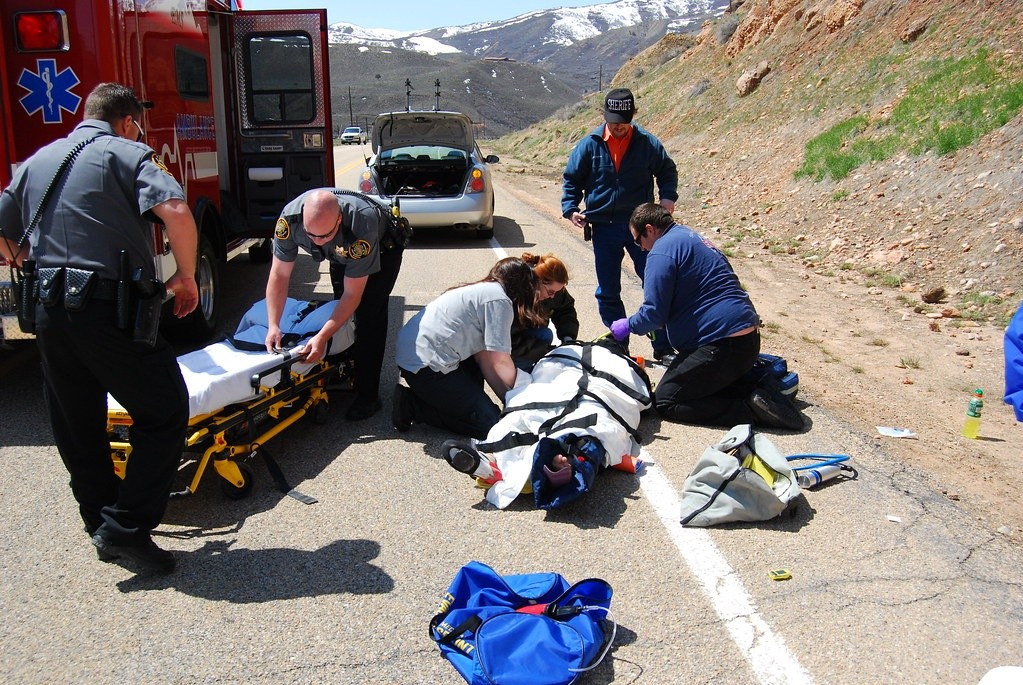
[678,424,802,528]
[428,560,618,685]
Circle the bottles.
[961,390,984,440]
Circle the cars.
[340,126,367,146]
[359,110,500,241]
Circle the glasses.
[633,227,646,248]
[120,111,145,142]
[541,284,559,297]
[302,208,342,239]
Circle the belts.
[90,277,119,301]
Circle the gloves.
[609,318,631,341]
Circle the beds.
[106,296,357,420]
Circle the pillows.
[234,296,357,336]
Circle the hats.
[603,87,635,124]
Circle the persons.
[396,257,544,441]
[0,82,198,574]
[561,88,680,365]
[265,187,404,421]
[510,252,578,374]
[610,202,805,430]
[1003,300,1023,422]
[441,339,652,511]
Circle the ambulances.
[0,1,337,355]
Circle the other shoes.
[746,388,806,430]
[653,348,678,366]
[391,382,412,433]
[344,397,385,422]
[90,533,177,570]
